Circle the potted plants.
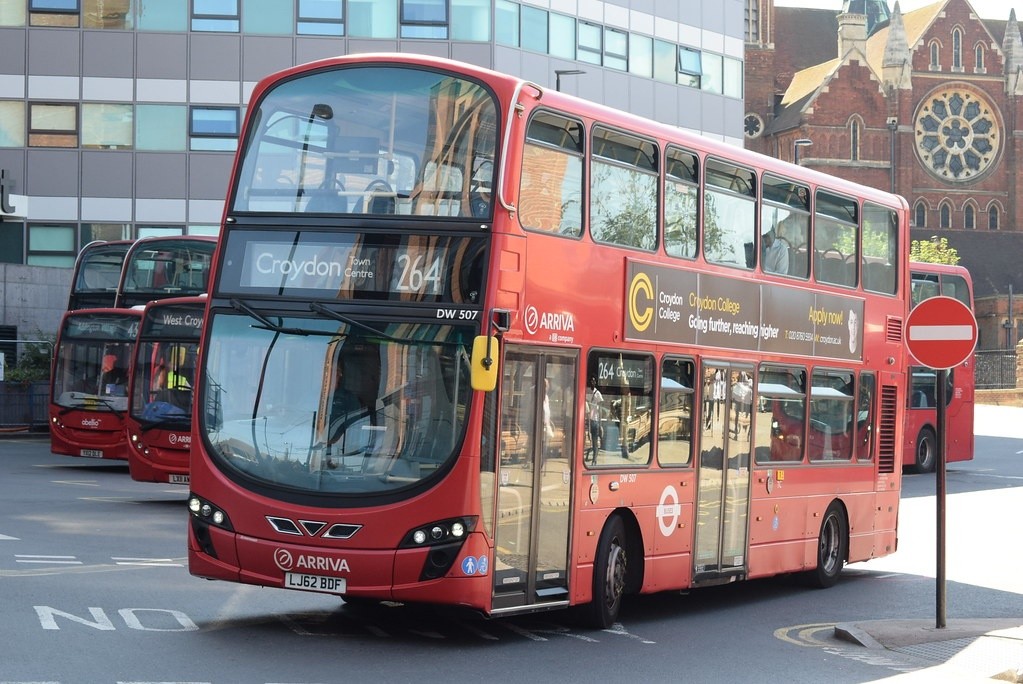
[0,328,59,427]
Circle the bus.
[48,235,219,504]
[767,243,976,475]
[183,51,912,630]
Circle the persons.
[763,222,790,276]
[586,375,605,465]
[707,368,751,442]
[523,375,555,471]
[295,356,361,423]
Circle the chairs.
[911,390,928,407]
[268,174,896,294]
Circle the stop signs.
[903,295,980,371]
